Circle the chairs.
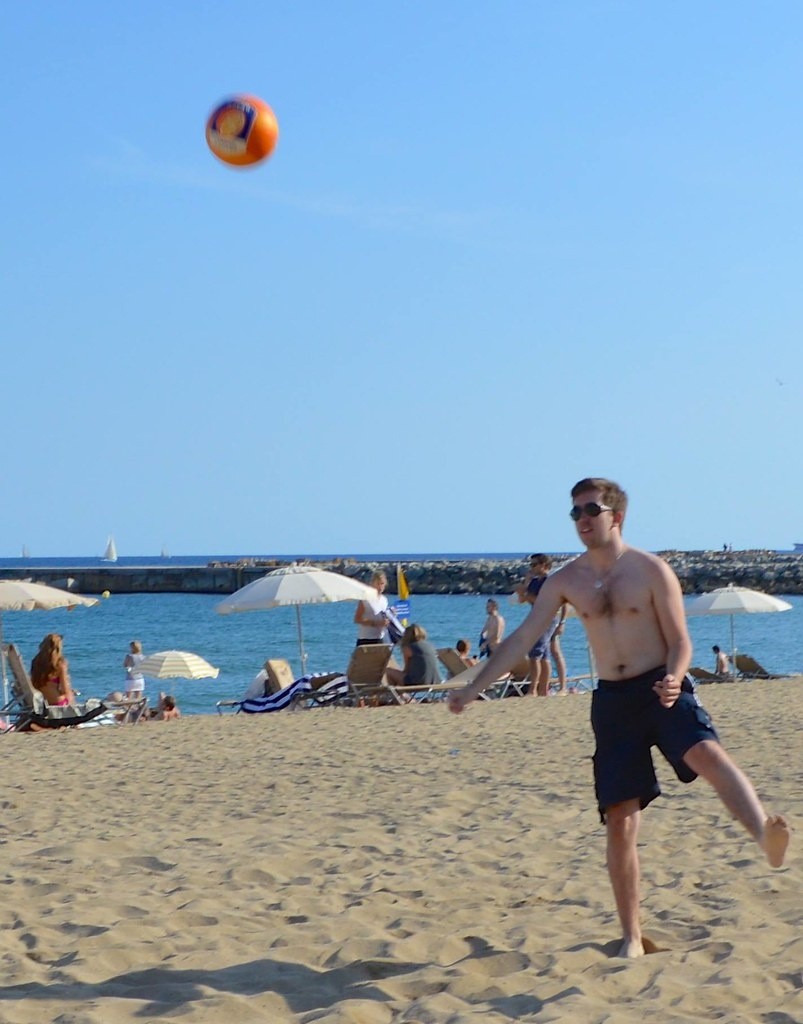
[689,667,733,684]
[215,642,598,716]
[726,654,790,680]
[0,643,107,734]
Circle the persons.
[481,598,505,658]
[456,640,477,667]
[448,478,789,958]
[387,624,440,704]
[123,641,145,697]
[354,571,396,647]
[108,691,179,722]
[711,645,729,682]
[518,553,567,697]
[31,634,79,730]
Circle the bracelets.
[559,621,565,624]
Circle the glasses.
[530,563,537,568]
[570,504,613,521]
[488,599,493,602]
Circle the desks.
[103,697,151,728]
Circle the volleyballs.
[206,94,278,166]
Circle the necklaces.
[585,551,623,589]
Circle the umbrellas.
[0,579,99,733]
[131,649,219,693]
[216,562,377,676]
[686,583,792,683]
[549,554,596,689]
